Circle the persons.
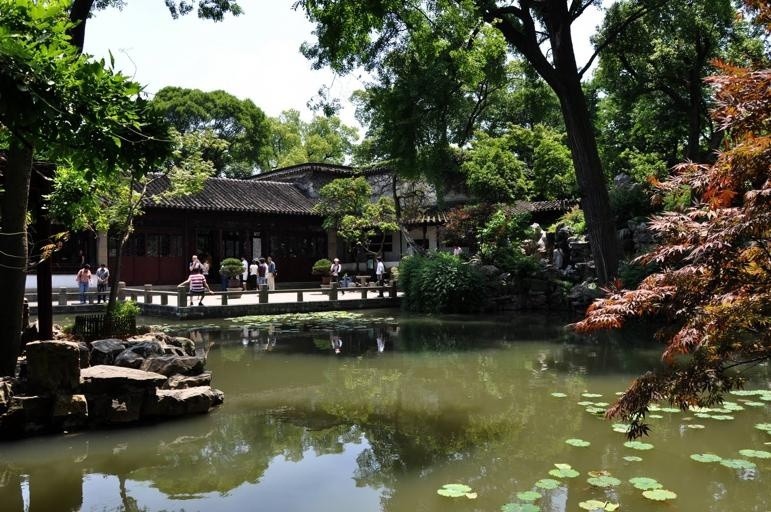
[95,264,110,303]
[177,268,214,307]
[376,257,385,297]
[330,257,345,295]
[75,263,92,303]
[454,244,463,256]
[188,255,277,293]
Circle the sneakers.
[191,302,193,305]
[199,303,203,306]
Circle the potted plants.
[220,257,245,287]
[311,258,333,284]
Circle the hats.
[334,258,340,262]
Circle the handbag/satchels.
[383,272,390,279]
[274,271,278,277]
